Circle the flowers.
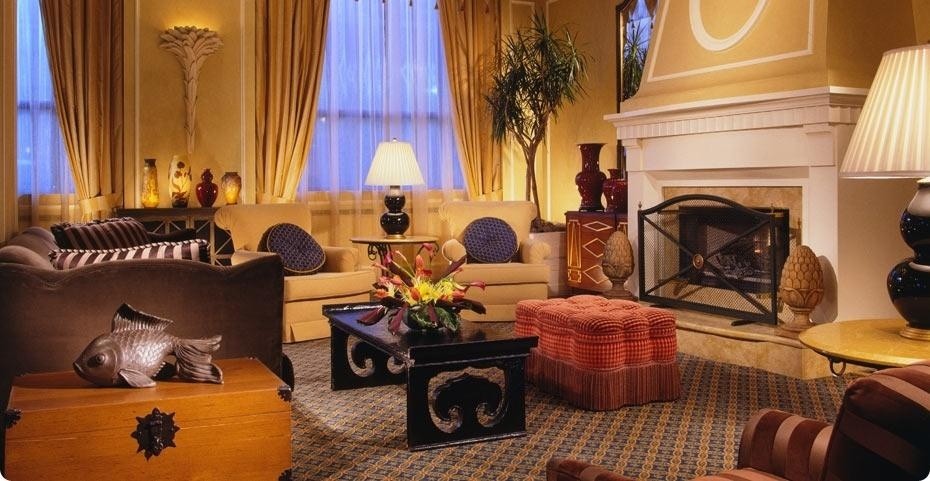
[357,243,489,333]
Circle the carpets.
[282,322,872,480]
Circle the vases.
[401,307,460,331]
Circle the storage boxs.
[4,355,294,481]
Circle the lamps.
[836,43,929,341]
[365,137,425,239]
[161,24,222,154]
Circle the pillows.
[464,216,517,264]
[49,238,211,270]
[51,215,151,250]
[264,221,325,276]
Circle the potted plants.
[460,17,597,298]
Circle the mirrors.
[615,0,653,169]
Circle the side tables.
[798,317,929,393]
[349,235,438,283]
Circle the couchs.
[514,294,679,412]
[1,226,285,380]
[545,361,930,480]
[432,200,554,322]
[213,204,376,342]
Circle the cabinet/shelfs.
[114,209,234,265]
[563,211,627,294]
